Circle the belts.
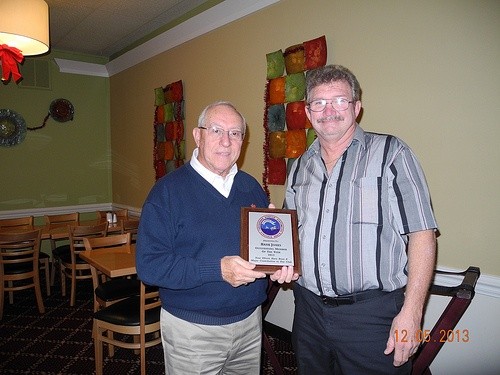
[319,291,370,308]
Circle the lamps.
[0,0,51,59]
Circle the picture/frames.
[240,206,303,276]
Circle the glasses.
[198,126,245,139]
[307,97,353,113]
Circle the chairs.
[121,217,140,244]
[0,215,51,297]
[90,280,163,375]
[0,225,46,319]
[82,232,160,356]
[95,209,129,220]
[59,221,110,306]
[43,211,87,294]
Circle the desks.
[78,243,137,278]
[46,215,140,290]
[0,225,51,240]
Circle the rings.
[244,283,248,285]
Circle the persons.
[134,102,275,375]
[269,64,437,375]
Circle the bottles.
[112,211,118,223]
[105,211,113,224]
